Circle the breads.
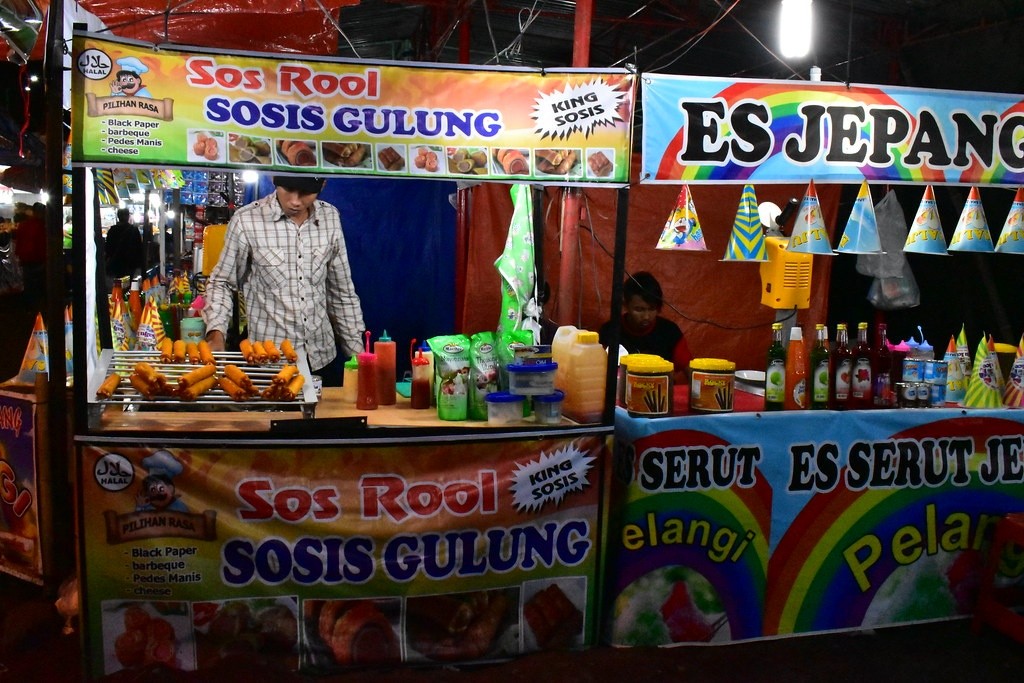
[535,150,577,175]
[192,133,218,161]
[415,146,438,172]
[113,607,180,668]
[229,136,270,163]
[321,141,367,167]
[525,584,585,651]
[195,604,298,677]
[588,151,613,177]
[447,148,488,175]
[406,587,516,661]
[378,147,405,172]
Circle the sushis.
[304,600,402,668]
[492,149,529,175]
[275,139,316,167]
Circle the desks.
[605,387,1024,650]
[75,387,612,683]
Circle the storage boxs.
[486,362,564,426]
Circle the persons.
[107,210,146,278]
[14,201,48,294]
[595,272,693,378]
[201,175,366,387]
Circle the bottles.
[343,327,436,415]
[763,320,889,412]
[551,323,607,422]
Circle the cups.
[179,318,205,342]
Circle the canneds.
[895,356,949,408]
